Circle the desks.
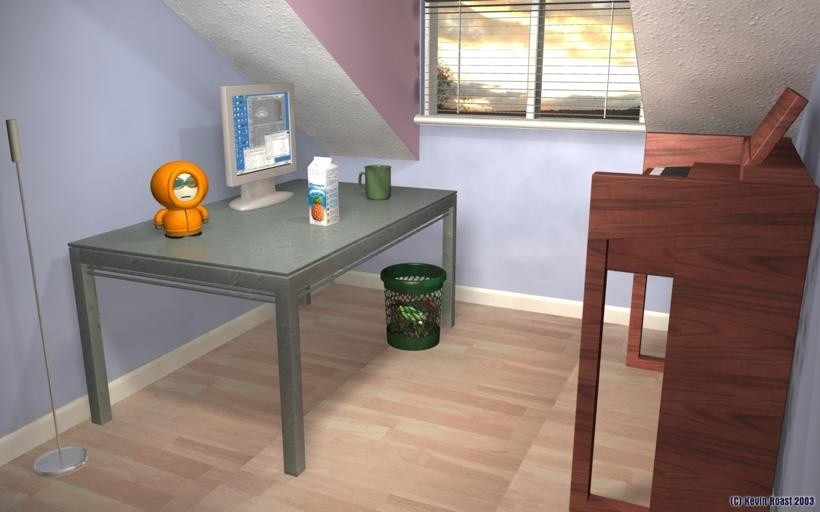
[68,177,458,477]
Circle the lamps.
[5,117,87,479]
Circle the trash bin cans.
[381,263,446,351]
